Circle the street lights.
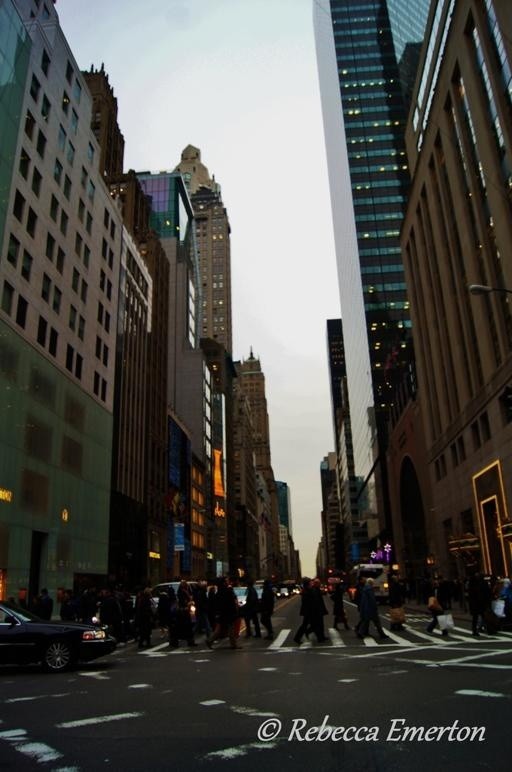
[469,284,509,297]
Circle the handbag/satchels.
[389,607,407,624]
[491,599,506,619]
[427,596,442,612]
[436,612,456,631]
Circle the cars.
[0,600,117,669]
[152,579,291,622]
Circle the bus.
[349,564,390,604]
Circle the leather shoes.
[116,623,499,649]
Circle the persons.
[292,569,512,645]
[7,574,275,649]
